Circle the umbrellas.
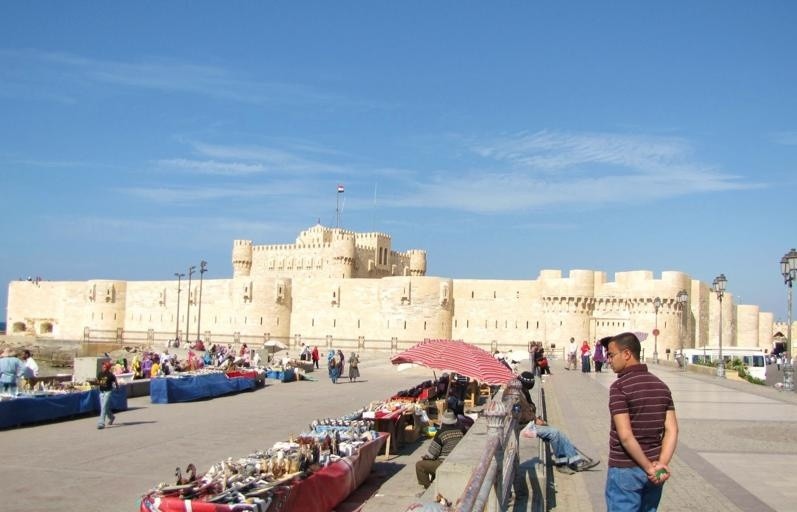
[391,337,513,409]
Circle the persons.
[593,338,606,372]
[111,338,360,385]
[580,341,592,371]
[435,373,476,433]
[764,349,788,372]
[416,410,464,490]
[604,332,678,512]
[516,371,601,475]
[495,341,553,379]
[565,337,579,370]
[87,362,119,428]
[19,349,39,392]
[0,348,26,392]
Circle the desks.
[149,360,265,403]
[350,376,466,453]
[0,358,149,430]
[140,430,391,510]
[267,369,298,383]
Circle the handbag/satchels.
[537,357,548,368]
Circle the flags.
[337,184,345,193]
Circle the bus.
[674,346,766,383]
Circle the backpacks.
[351,357,357,368]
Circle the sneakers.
[556,464,576,475]
[575,459,601,471]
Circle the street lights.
[651,296,660,365]
[676,290,687,372]
[779,248,796,388]
[185,265,196,344]
[173,272,184,341]
[197,260,209,349]
[711,273,727,377]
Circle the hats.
[517,371,537,390]
[1,347,17,357]
[101,361,111,369]
[438,408,458,425]
